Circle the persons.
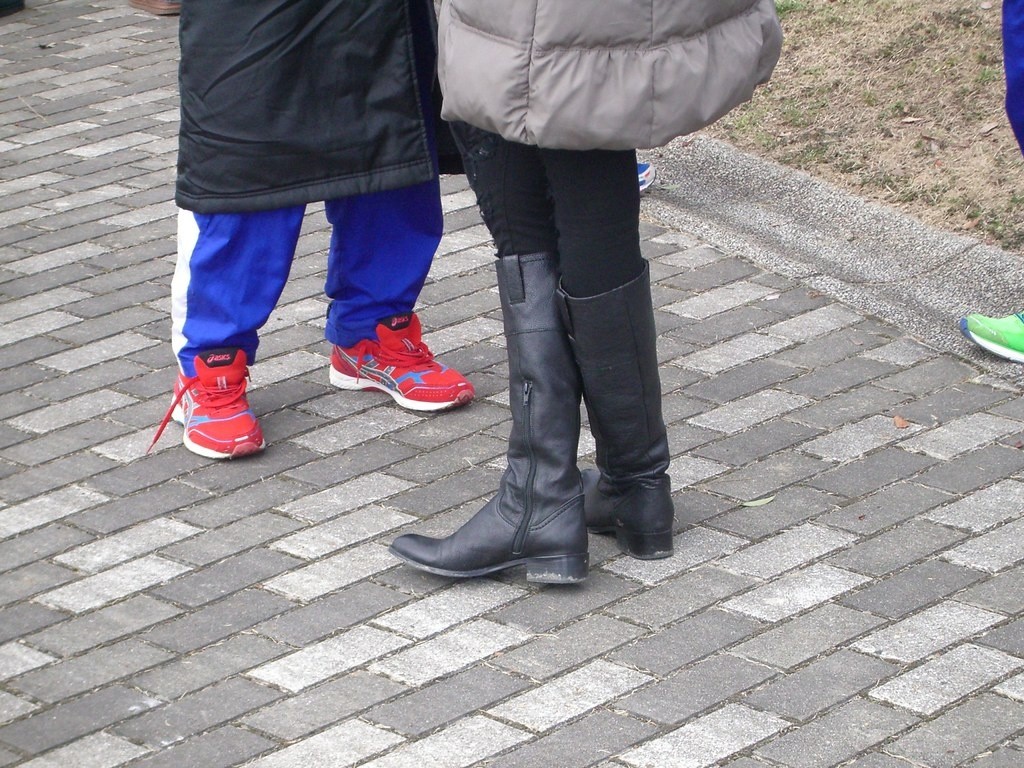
[0,1,26,19]
[172,1,475,461]
[960,0,1024,362]
[638,162,655,197]
[129,0,181,15]
[389,0,783,584]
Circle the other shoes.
[0,0,25,16]
[636,164,656,192]
[128,0,181,15]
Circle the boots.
[389,248,588,584]
[555,255,674,559]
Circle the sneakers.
[329,312,475,411]
[146,349,265,459]
[960,310,1024,364]
